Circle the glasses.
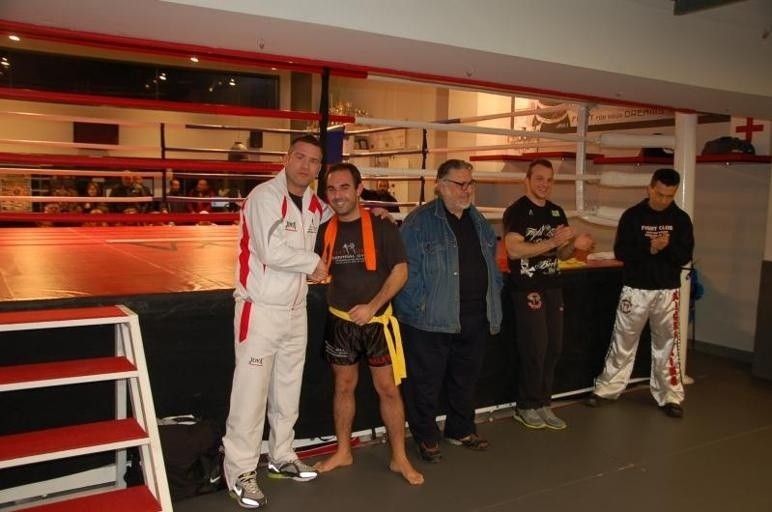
[445,178,476,191]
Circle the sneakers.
[419,441,443,464]
[512,406,546,430]
[268,460,319,482]
[585,393,619,406]
[447,433,490,452]
[536,404,568,431]
[229,470,269,509]
[662,399,685,418]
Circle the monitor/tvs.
[73,122,119,144]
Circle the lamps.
[226,62,252,164]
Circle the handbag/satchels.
[123,410,226,506]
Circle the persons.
[398,156,505,464]
[221,133,399,510]
[584,168,697,419]
[500,158,596,432]
[313,163,426,488]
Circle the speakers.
[251,131,262,147]
